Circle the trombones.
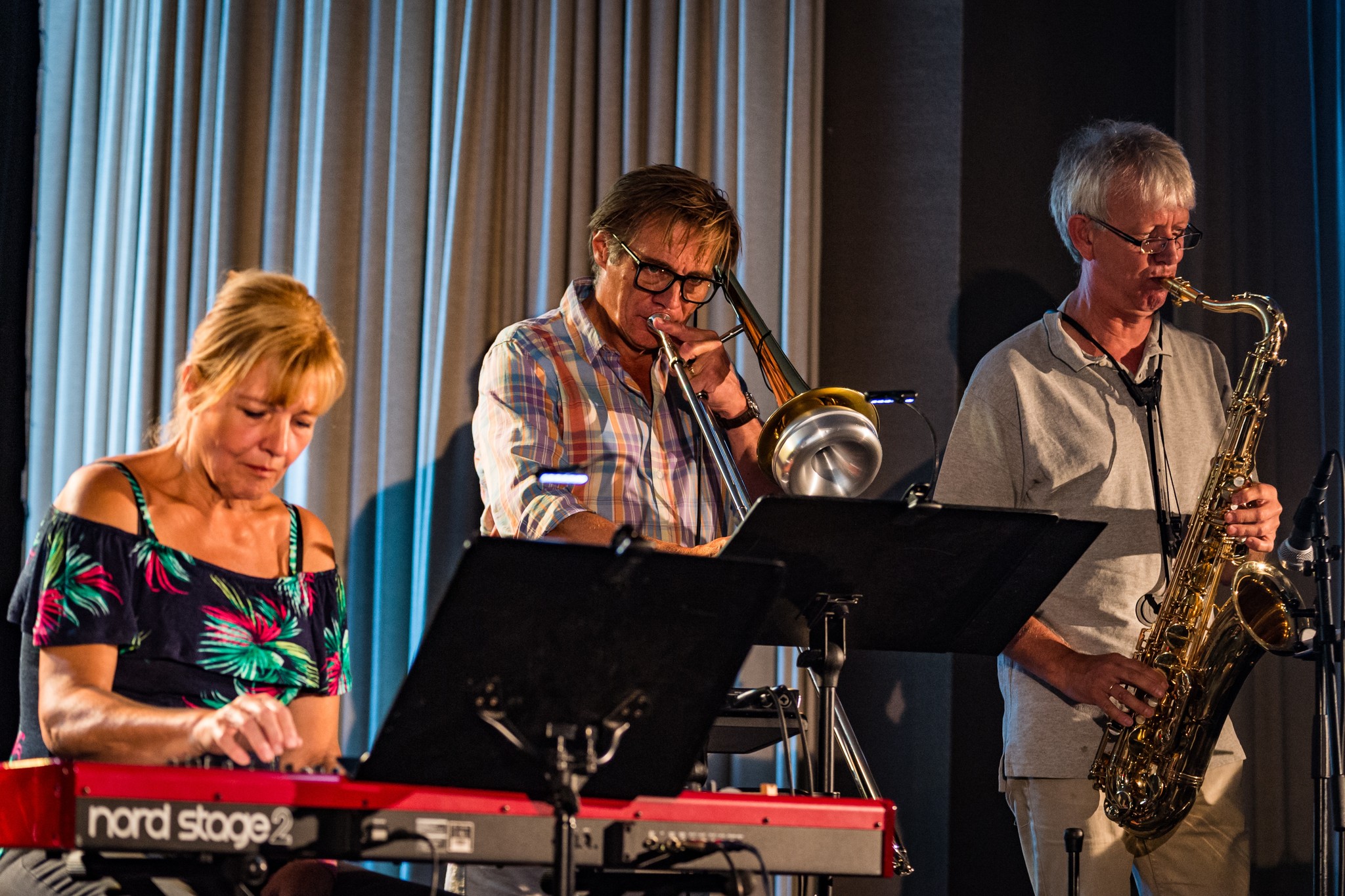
[644,265,913,876]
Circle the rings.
[1108,682,1120,696]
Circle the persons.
[927,122,1283,896]
[441,164,778,896]
[0,269,352,896]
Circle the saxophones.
[1090,275,1315,856]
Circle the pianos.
[0,758,897,895]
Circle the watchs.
[715,391,761,431]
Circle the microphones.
[1278,452,1336,573]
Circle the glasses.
[605,227,723,304]
[1075,212,1204,254]
